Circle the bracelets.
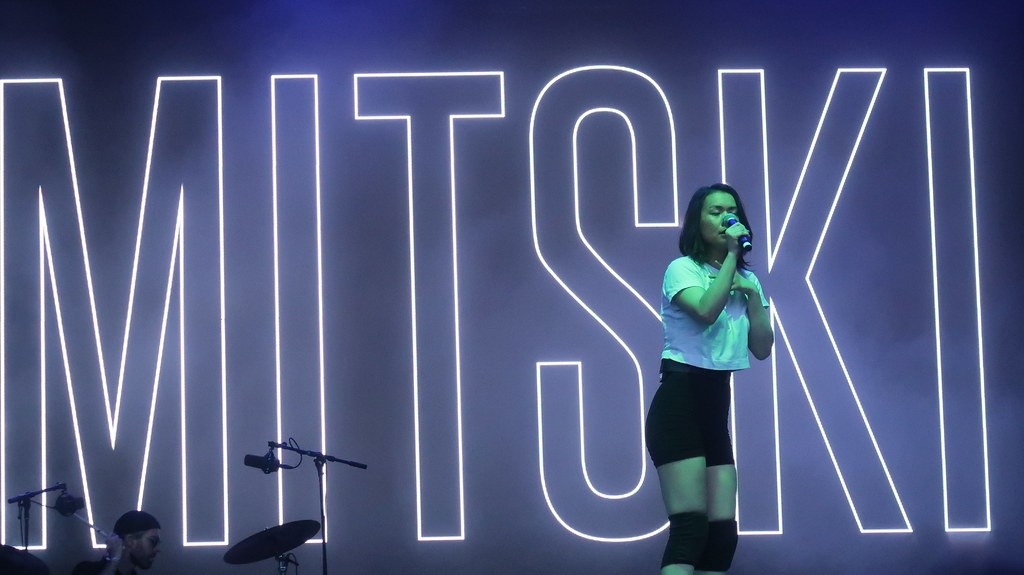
[112,557,121,562]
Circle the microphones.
[244,454,282,474]
[55,492,84,515]
[722,213,753,251]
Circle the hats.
[113,510,161,535]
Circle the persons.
[645,183,774,575]
[73,510,161,575]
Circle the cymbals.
[222,519,321,565]
[0,543,51,575]
